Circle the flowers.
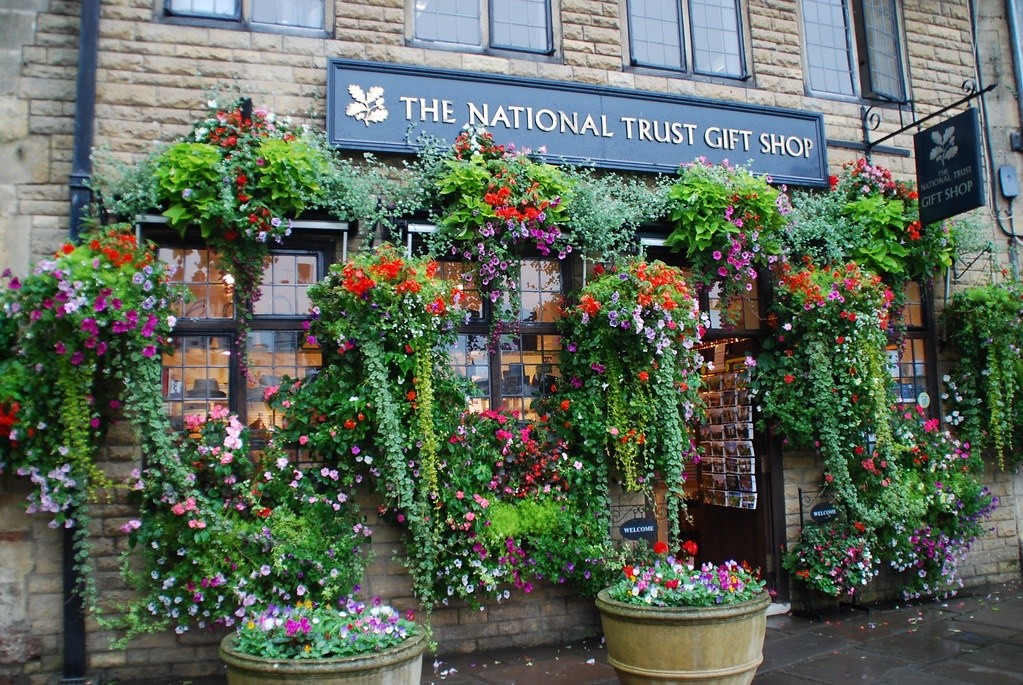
[0,98,1023,685]
